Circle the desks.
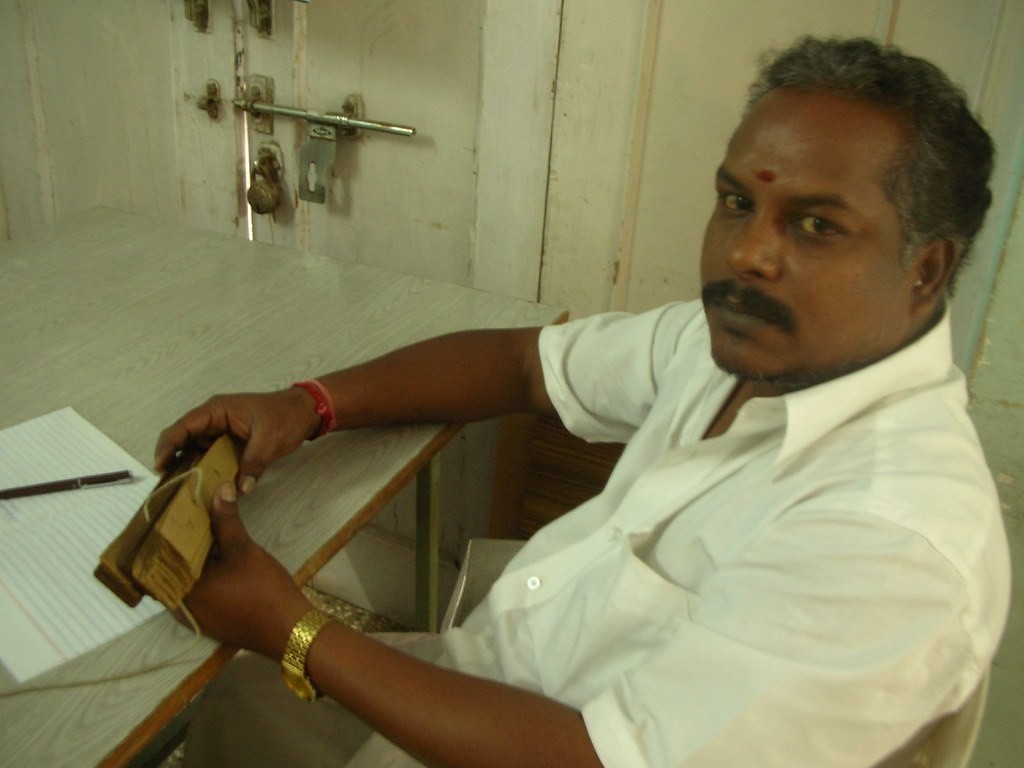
[0,206,571,768]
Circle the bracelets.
[292,377,338,442]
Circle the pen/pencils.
[0,470,132,500]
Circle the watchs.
[279,607,346,705]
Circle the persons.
[153,36,1024,768]
